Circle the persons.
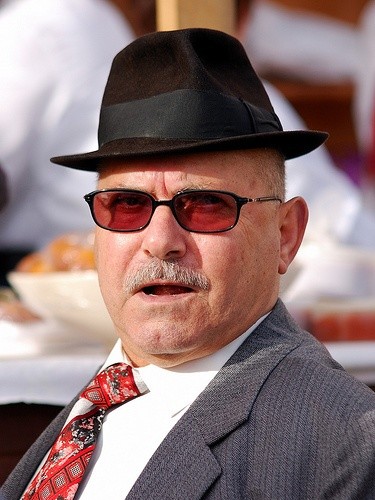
[0,0,138,274]
[0,25,374,499]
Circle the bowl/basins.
[9,263,305,346]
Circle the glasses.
[82,185,283,236]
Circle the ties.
[15,362,149,500]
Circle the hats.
[49,27,329,173]
